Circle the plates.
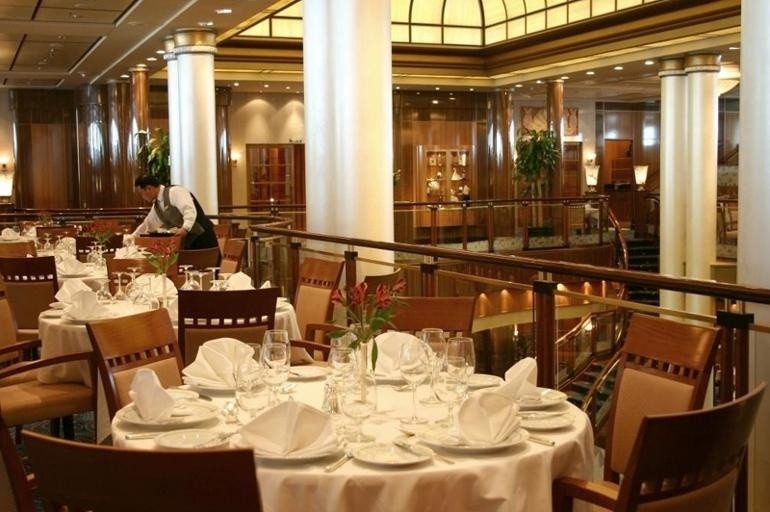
[350,441,436,466]
[516,409,575,430]
[233,433,346,463]
[467,372,503,387]
[154,429,231,449]
[48,300,69,310]
[414,424,529,452]
[115,399,222,429]
[483,386,568,411]
[290,364,327,382]
[61,311,88,326]
[182,377,236,396]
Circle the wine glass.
[145,271,160,311]
[431,355,468,427]
[132,275,151,314]
[205,266,222,291]
[94,278,112,306]
[186,268,200,291]
[178,262,193,291]
[95,251,107,273]
[340,367,378,446]
[233,343,261,378]
[54,234,64,247]
[444,336,476,380]
[126,265,142,273]
[97,244,106,258]
[264,328,290,371]
[327,348,358,423]
[258,344,290,404]
[90,240,100,250]
[194,271,211,291]
[87,245,96,263]
[43,232,52,237]
[127,272,139,298]
[112,271,132,315]
[420,327,444,355]
[42,237,52,251]
[400,343,430,424]
[235,364,267,417]
[208,277,226,292]
[218,272,233,291]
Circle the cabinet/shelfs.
[244,143,306,230]
[516,140,584,232]
[412,145,478,227]
[602,139,634,188]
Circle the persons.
[126,173,226,282]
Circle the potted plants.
[509,126,566,240]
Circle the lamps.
[0,155,9,171]
[633,164,649,193]
[583,162,603,194]
[585,152,598,166]
